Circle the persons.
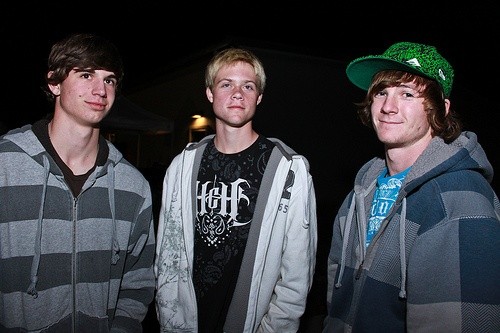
[0,33,157,333]
[323,41,500,333]
[153,46,318,333]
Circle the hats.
[345,41,455,99]
